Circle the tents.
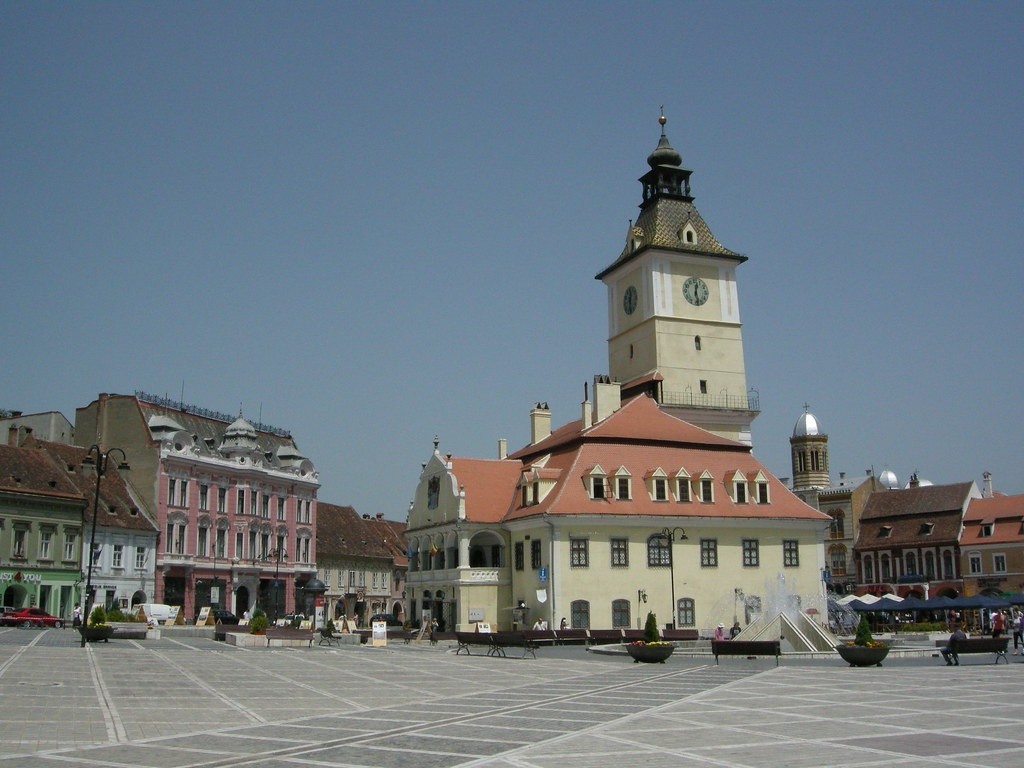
[827,593,1024,632]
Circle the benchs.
[939,636,1009,665]
[266,629,315,649]
[353,630,412,645]
[663,629,699,640]
[215,624,249,640]
[711,640,781,667]
[625,630,661,642]
[105,622,148,641]
[431,629,623,660]
[319,627,341,647]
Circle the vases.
[625,642,679,663]
[832,644,891,668]
[76,626,117,643]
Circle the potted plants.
[249,607,270,635]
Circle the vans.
[133,604,187,626]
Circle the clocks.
[622,285,637,314]
[683,277,708,306]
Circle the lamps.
[638,589,648,604]
[735,587,745,600]
[822,562,831,583]
[195,578,202,585]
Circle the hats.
[718,623,724,628]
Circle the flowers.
[83,605,112,628]
[638,613,669,645]
[842,612,888,647]
[629,641,672,646]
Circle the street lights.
[656,527,688,629]
[78,444,131,647]
[266,547,289,621]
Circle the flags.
[407,544,414,561]
[431,542,439,555]
[416,541,421,568]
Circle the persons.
[243,609,249,618]
[432,618,438,633]
[68,603,83,627]
[560,617,569,629]
[715,623,725,640]
[532,617,547,630]
[940,608,1024,667]
[729,622,741,639]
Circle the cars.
[194,610,240,625]
[369,614,403,627]
[0,607,65,628]
[270,614,305,626]
[0,606,14,617]
[871,612,899,623]
